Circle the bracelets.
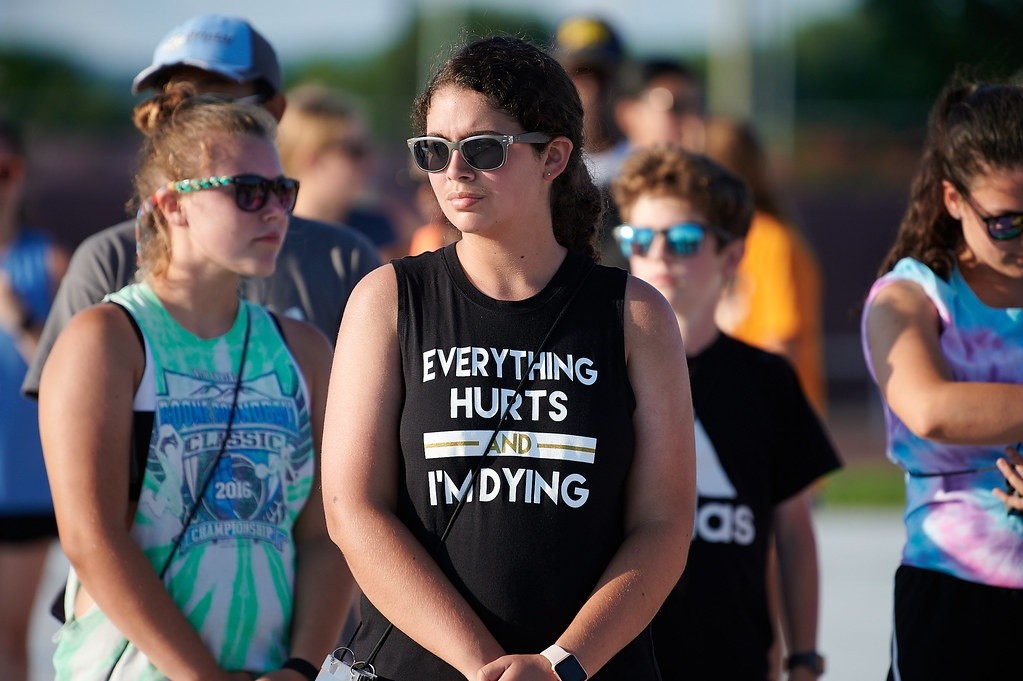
[280,657,320,681]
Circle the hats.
[134,14,282,93]
[554,17,622,91]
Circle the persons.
[0,119,74,681]
[860,72,1023,681]
[36,81,354,681]
[615,143,848,681]
[276,20,825,681]
[320,34,699,681]
[20,13,386,401]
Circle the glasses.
[0,166,13,182]
[407,132,553,172]
[611,221,735,258]
[942,164,1023,240]
[170,174,300,215]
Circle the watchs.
[540,645,589,681]
[783,652,824,673]
